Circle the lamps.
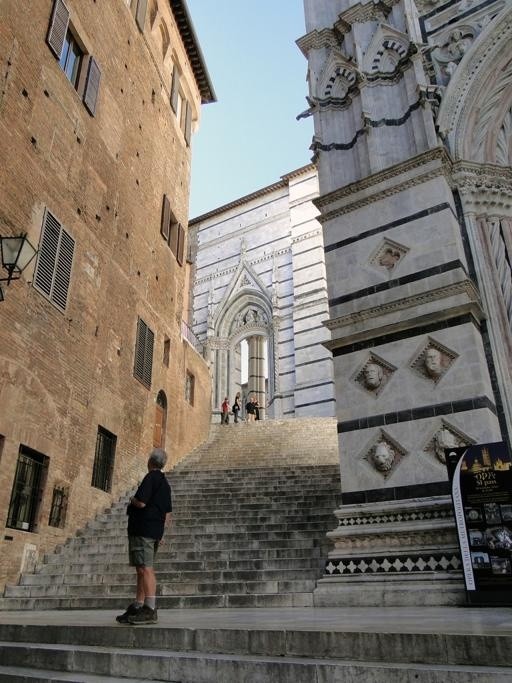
[0,231,38,302]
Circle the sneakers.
[115,604,144,624]
[128,605,159,624]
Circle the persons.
[245,396,259,422]
[232,391,242,422]
[115,448,173,626]
[220,396,233,424]
